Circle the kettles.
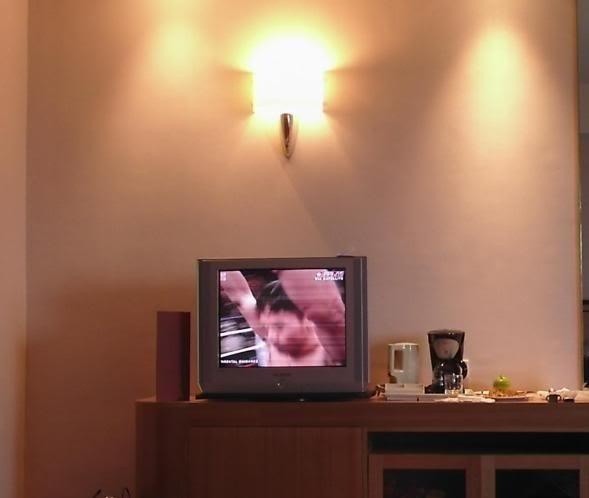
[388,342,420,384]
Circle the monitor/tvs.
[197,255,368,398]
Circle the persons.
[220,269,346,367]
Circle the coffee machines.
[425,328,467,394]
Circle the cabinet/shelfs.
[134,393,589,498]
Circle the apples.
[493,376,510,392]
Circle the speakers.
[157,311,190,401]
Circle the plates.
[491,395,529,400]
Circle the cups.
[443,374,463,397]
